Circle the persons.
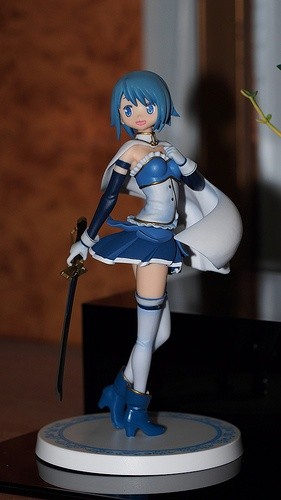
[65,68,206,439]
